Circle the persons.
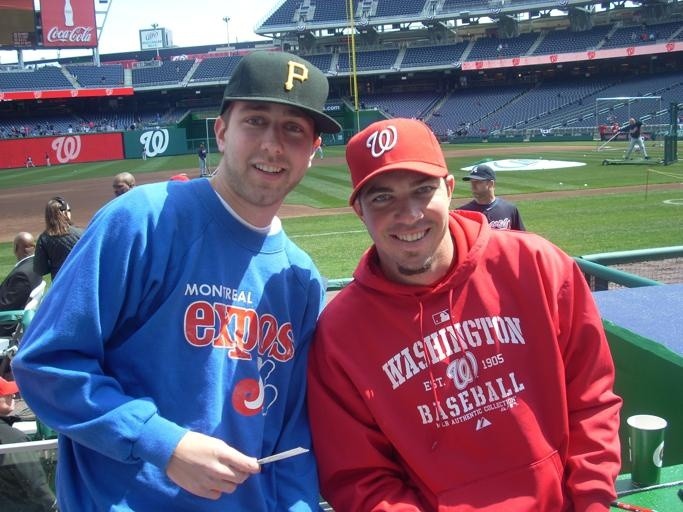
[303,118,626,511]
[24,155,35,169]
[0,231,45,337]
[0,110,158,138]
[452,165,525,231]
[0,356,57,512]
[112,171,134,197]
[44,152,51,167]
[195,141,206,178]
[8,49,331,511]
[622,118,652,161]
[629,30,660,42]
[30,197,86,296]
[598,109,620,142]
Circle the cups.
[627,414,668,488]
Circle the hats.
[344,117,447,207]
[461,165,496,182]
[0,377,18,398]
[217,50,342,135]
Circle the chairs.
[0,289,44,378]
[0,280,47,336]
